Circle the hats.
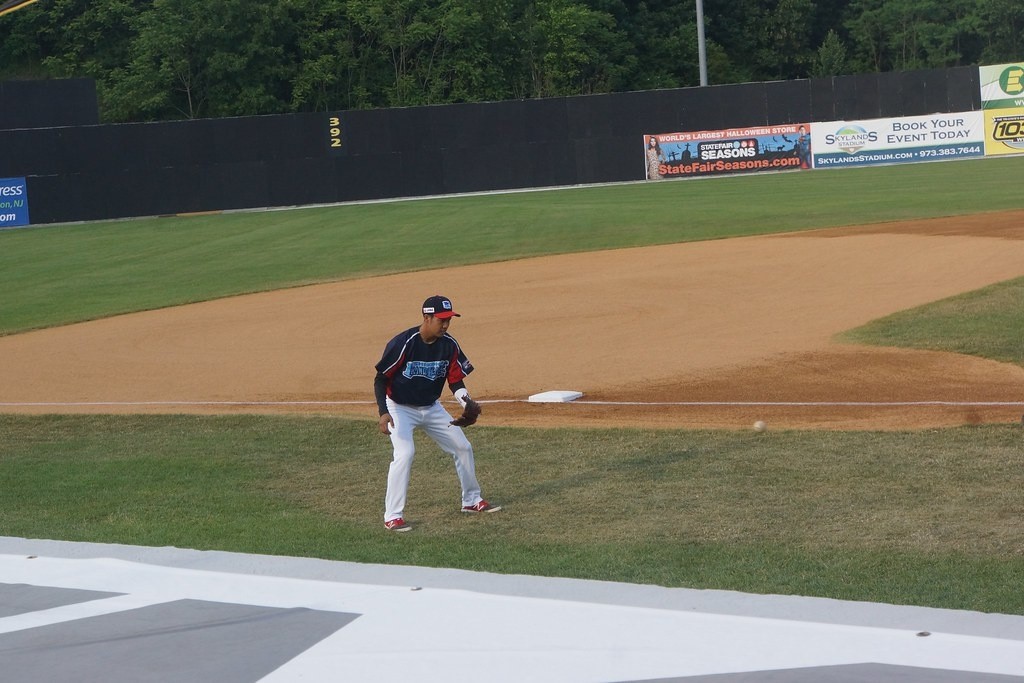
[421,294,461,319]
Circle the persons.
[374,295,501,532]
[646,137,666,179]
[793,126,812,169]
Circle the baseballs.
[754,421,767,433]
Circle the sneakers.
[384,518,412,533]
[460,500,502,514]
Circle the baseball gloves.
[448,395,481,428]
